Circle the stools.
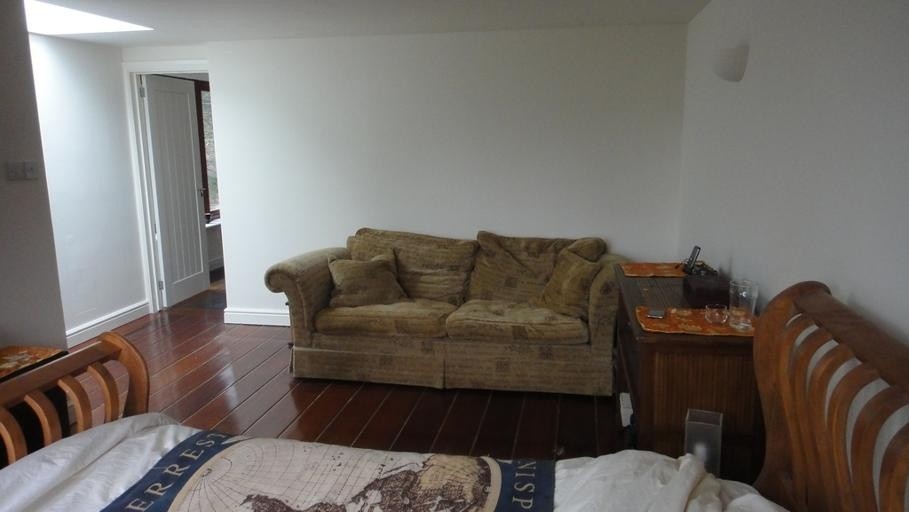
[0,345,71,467]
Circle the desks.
[614,260,767,486]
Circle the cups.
[729,278,759,331]
[706,303,728,324]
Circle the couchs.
[263,227,628,398]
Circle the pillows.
[324,249,408,308]
[530,250,605,324]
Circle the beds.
[1,282,909,510]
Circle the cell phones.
[686,246,701,269]
[648,310,664,319]
